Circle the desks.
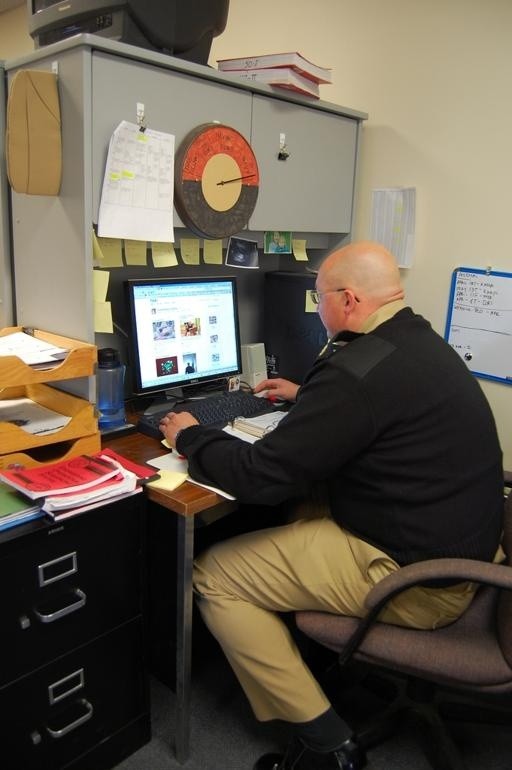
[102,405,268,763]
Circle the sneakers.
[256,731,367,770]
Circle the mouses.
[252,390,285,407]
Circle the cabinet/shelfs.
[0,481,155,769]
[5,32,368,408]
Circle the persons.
[153,321,175,340]
[183,321,198,337]
[185,361,195,375]
[273,235,290,254]
[157,240,504,769]
[268,232,280,254]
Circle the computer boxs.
[239,271,329,389]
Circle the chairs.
[297,470,510,769]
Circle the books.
[0,329,163,534]
[221,408,288,448]
[221,70,321,100]
[215,52,333,88]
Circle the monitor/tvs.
[28,0,230,65]
[123,274,243,416]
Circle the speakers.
[241,343,268,392]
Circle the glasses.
[310,288,360,305]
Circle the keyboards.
[138,388,278,439]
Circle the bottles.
[97,348,127,430]
